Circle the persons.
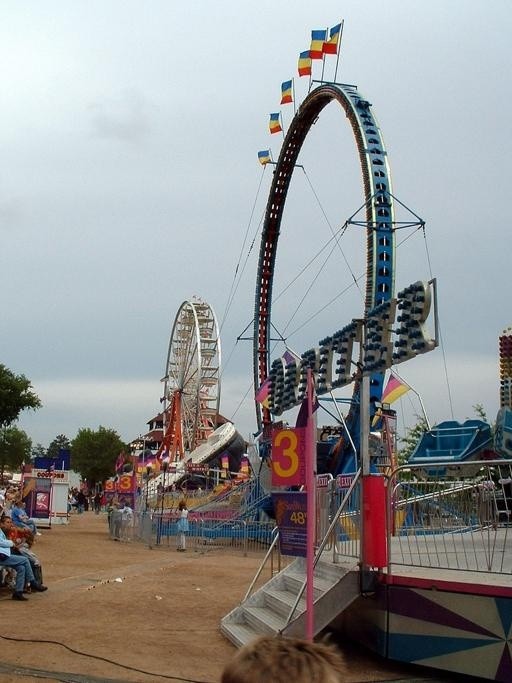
[156,482,165,505]
[175,501,190,553]
[219,634,348,683]
[171,481,178,492]
[483,467,511,525]
[67,484,135,542]
[0,478,48,602]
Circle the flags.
[281,77,296,104]
[321,18,344,54]
[257,147,275,166]
[308,26,329,61]
[372,367,411,428]
[254,345,302,413]
[267,110,284,135]
[297,49,313,78]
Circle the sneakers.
[11,593,28,601]
[30,582,48,593]
